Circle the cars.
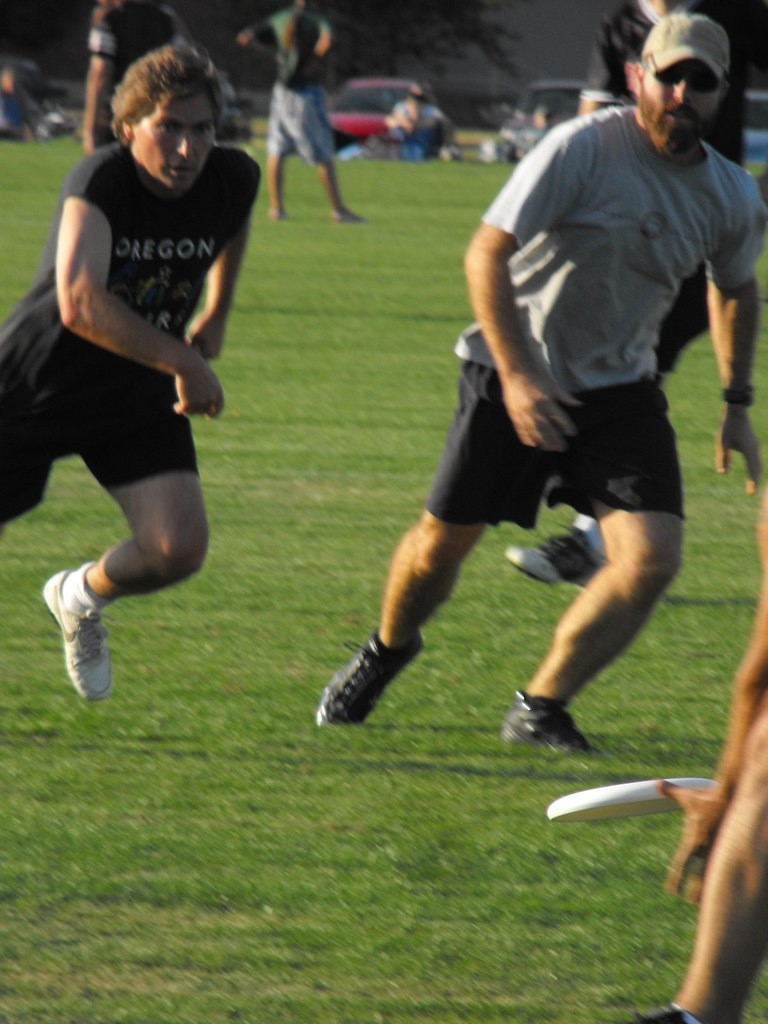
[322,76,439,150]
[736,87,768,170]
[489,80,623,164]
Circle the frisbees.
[546,776,718,822]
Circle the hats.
[642,12,730,83]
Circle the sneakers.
[500,690,601,757]
[42,570,112,701]
[505,523,600,588]
[316,630,424,730]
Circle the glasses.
[655,71,719,92]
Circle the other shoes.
[618,1005,693,1024]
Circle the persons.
[615,489,767,1024]
[515,105,550,160]
[81,0,180,157]
[505,0,768,593]
[314,14,768,751]
[389,84,456,161]
[236,0,364,224]
[0,48,260,702]
[0,57,48,140]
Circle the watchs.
[721,385,752,405]
